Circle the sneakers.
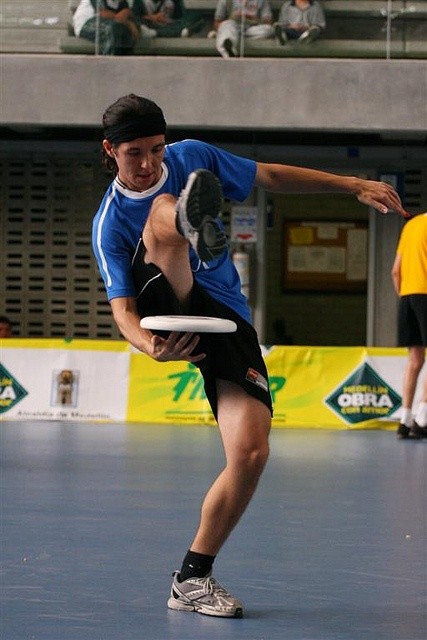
[167,569,244,617]
[223,39,234,57]
[396,423,410,436]
[301,28,318,43]
[408,419,427,438]
[174,168,228,261]
[275,25,287,45]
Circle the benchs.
[59,0,426,59]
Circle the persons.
[90,94,411,618]
[391,213,427,437]
[208,0,276,58]
[73,0,122,56]
[132,0,207,39]
[97,1,141,55]
[274,0,327,45]
[0,319,12,339]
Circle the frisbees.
[139,316,237,333]
